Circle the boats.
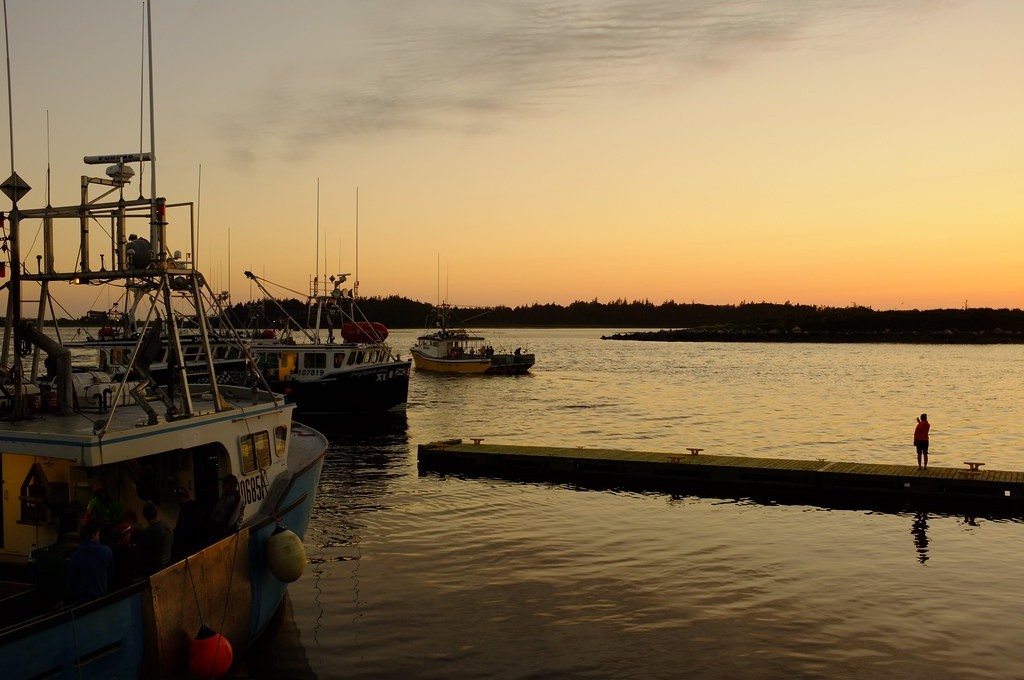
[43,162,415,425]
[479,354,536,376]
[1,54,330,680]
[407,253,492,375]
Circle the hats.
[219,474,237,484]
[92,482,104,491]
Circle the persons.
[514,347,522,355]
[913,413,931,470]
[470,347,475,355]
[480,346,494,357]
[69,473,244,600]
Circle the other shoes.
[918,466,922,470]
[924,467,928,470]
